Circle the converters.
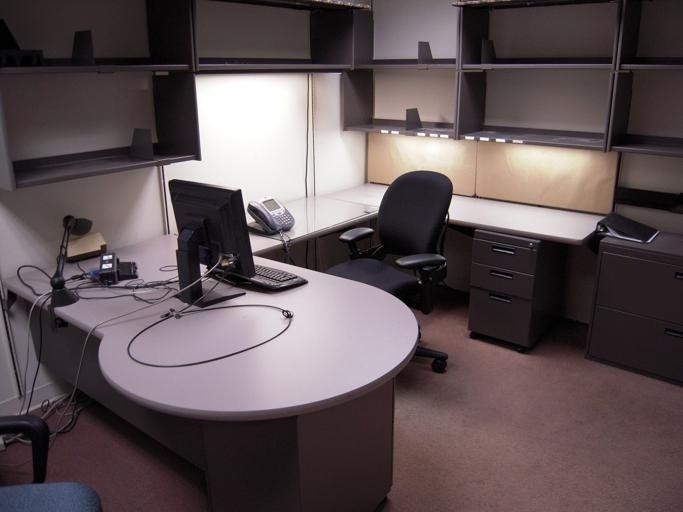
[100,252,118,284]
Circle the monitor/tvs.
[169,179,256,308]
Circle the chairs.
[0,415,105,512]
[323,169,455,374]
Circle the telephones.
[247,195,295,238]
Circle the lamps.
[48,212,91,307]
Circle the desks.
[3,182,605,512]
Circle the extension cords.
[0,435,6,451]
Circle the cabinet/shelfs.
[191,1,357,71]
[466,229,566,349]
[457,0,624,151]
[1,1,201,191]
[341,3,459,139]
[606,0,682,158]
[583,231,682,386]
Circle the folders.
[595,212,660,244]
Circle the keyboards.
[207,264,308,294]
